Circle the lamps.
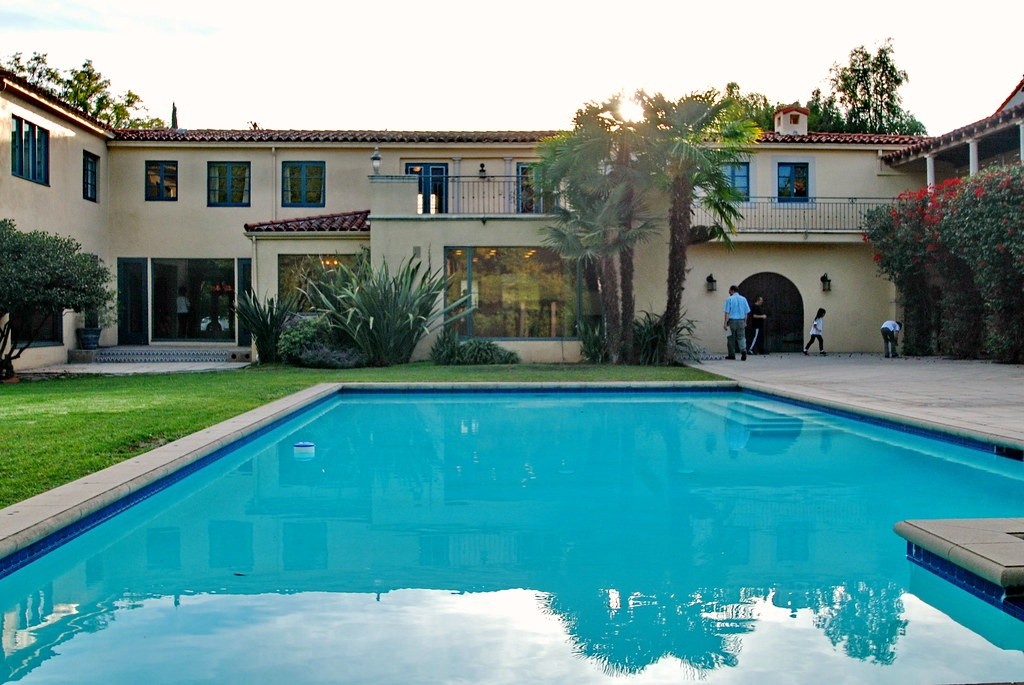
[370,149,382,175]
[821,273,831,291]
[478,163,486,178]
[706,274,716,290]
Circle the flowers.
[206,281,233,317]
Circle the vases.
[206,318,223,339]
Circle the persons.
[460,290,467,308]
[722,285,751,361]
[746,296,767,355]
[880,320,903,358]
[176,286,190,338]
[802,308,827,355]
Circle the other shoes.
[892,354,899,357]
[741,352,746,360]
[725,356,735,360]
[885,356,890,358]
[748,350,757,355]
[801,350,808,356]
[820,351,828,355]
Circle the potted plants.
[231,289,306,366]
[75,302,120,349]
